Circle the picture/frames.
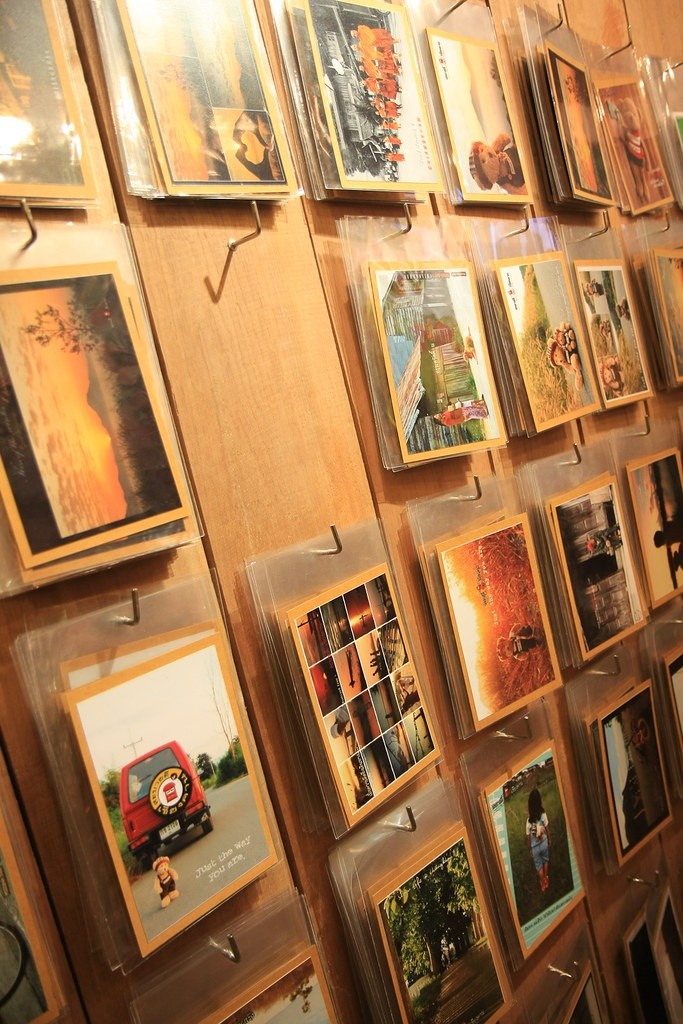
[1,1,683,1024]
[0,261,197,586]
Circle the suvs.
[119,740,214,870]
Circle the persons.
[349,25,405,163]
[586,524,623,553]
[330,704,350,738]
[431,394,489,428]
[526,789,553,892]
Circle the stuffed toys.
[546,322,583,391]
[619,96,653,203]
[585,279,605,297]
[153,856,179,907]
[616,298,631,321]
[599,357,625,391]
[496,622,538,661]
[468,134,528,195]
[600,319,611,334]
